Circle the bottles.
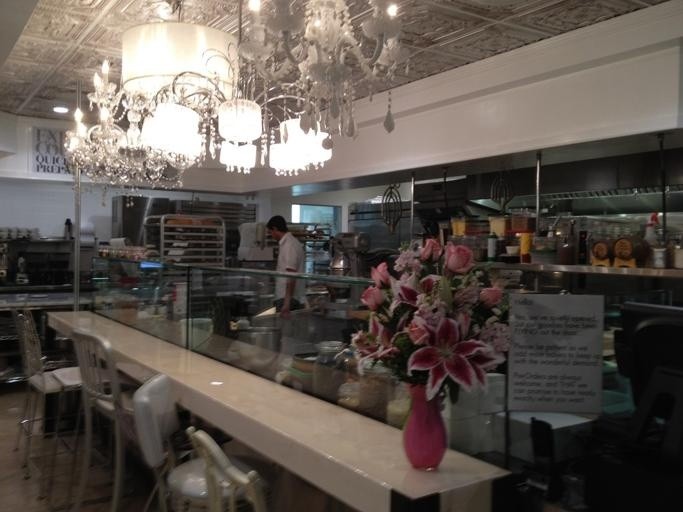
[652,229,668,269]
[313,340,347,406]
[98,244,147,263]
[64,218,72,241]
[486,231,499,258]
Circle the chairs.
[188,427,262,510]
[70,330,137,506]
[131,374,262,509]
[18,312,110,467]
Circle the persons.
[265,215,306,358]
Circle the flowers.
[359,237,512,406]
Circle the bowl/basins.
[506,246,520,255]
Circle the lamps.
[59,0,415,209]
